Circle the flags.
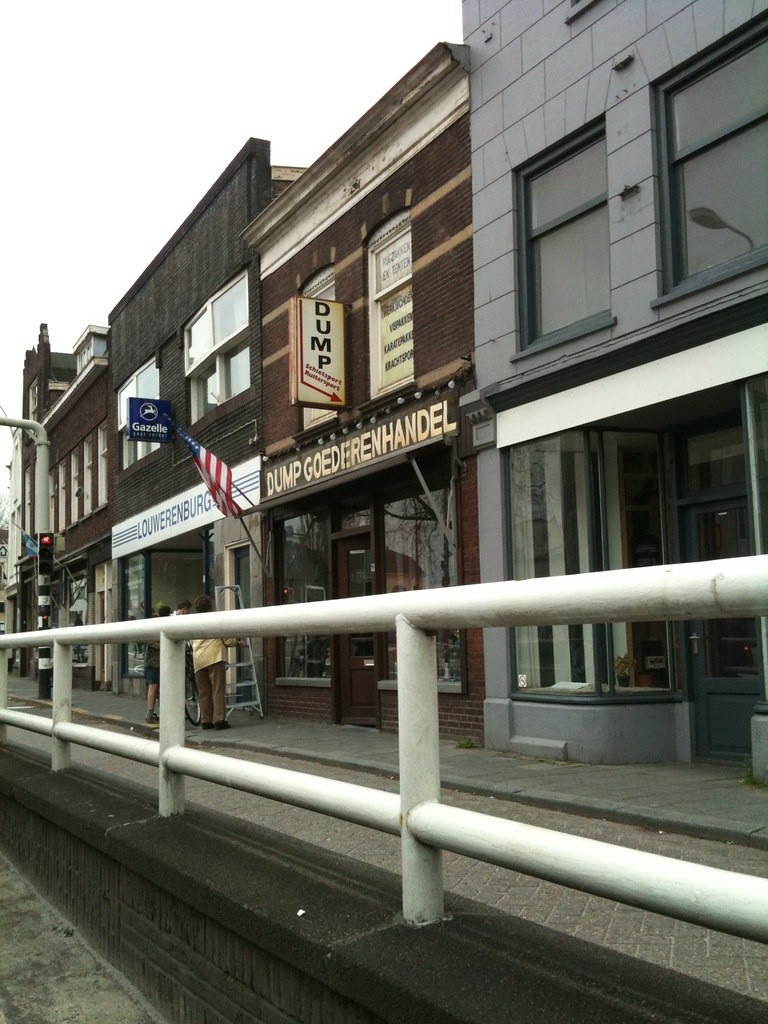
[21,531,56,563]
[163,411,245,520]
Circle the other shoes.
[202,722,215,729]
[214,720,231,729]
[144,713,159,723]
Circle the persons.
[144,596,192,724]
[188,593,247,731]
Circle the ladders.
[214,585,265,723]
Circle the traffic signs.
[0,544,8,563]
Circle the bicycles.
[179,642,203,727]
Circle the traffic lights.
[37,531,54,575]
